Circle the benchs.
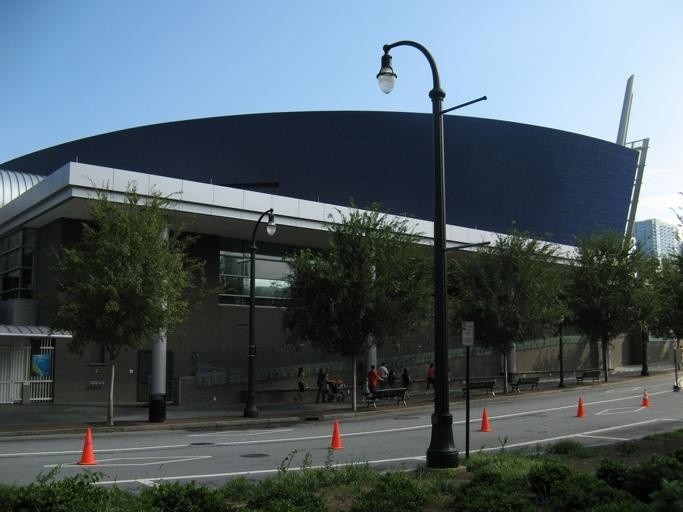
[508,377,540,394]
[461,380,496,399]
[366,388,409,409]
[575,370,601,385]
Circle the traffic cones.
[575,396,588,418]
[329,420,344,449]
[640,387,650,407]
[478,407,492,432]
[76,427,96,465]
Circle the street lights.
[377,40,460,472]
[243,208,278,403]
[669,327,679,392]
[555,313,567,387]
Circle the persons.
[401,366,410,400]
[293,366,306,402]
[387,367,395,400]
[367,364,385,409]
[314,366,326,402]
[377,362,388,388]
[425,363,435,395]
[324,366,330,391]
[361,375,369,403]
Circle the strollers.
[328,380,349,403]
